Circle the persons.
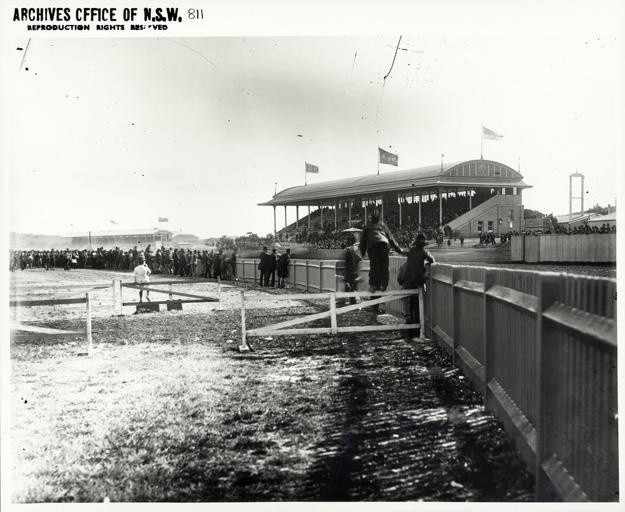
[9,191,616,340]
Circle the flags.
[481,127,504,141]
[305,162,319,174]
[379,147,399,167]
